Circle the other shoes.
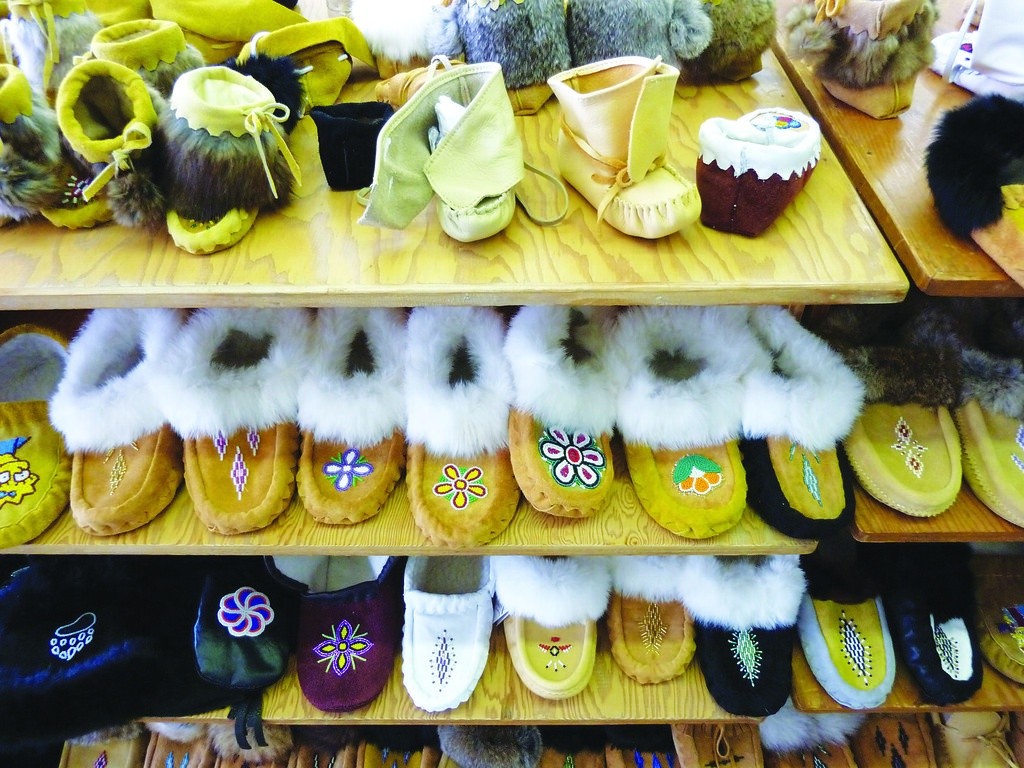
[695,107,822,237]
[0,555,1024,768]
[920,91,1024,288]
[425,0,571,117]
[566,0,714,71]
[0,306,1024,546]
[782,0,940,120]
[682,0,777,79]
[348,0,457,80]
[927,0,1024,102]
[308,101,395,190]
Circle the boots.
[0,0,378,255]
[357,62,570,242]
[546,55,702,239]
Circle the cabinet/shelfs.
[0,42,908,725]
[769,30,1024,712]
